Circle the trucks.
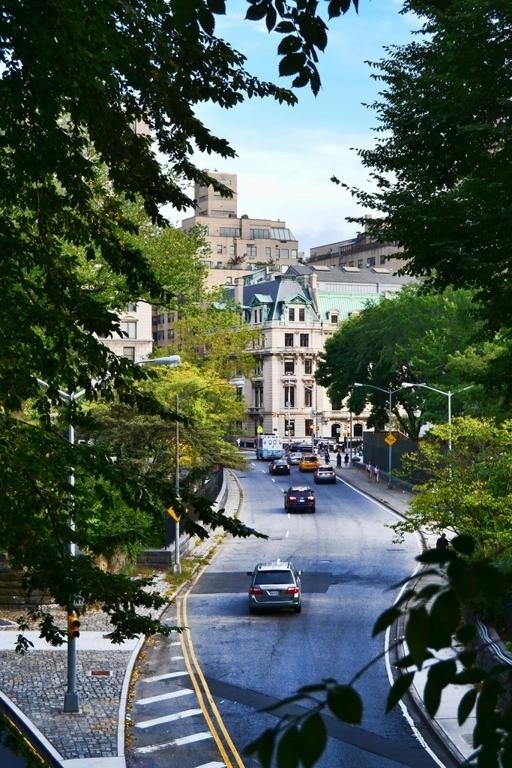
[254,432,285,460]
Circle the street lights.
[285,371,296,445]
[345,388,353,468]
[354,382,430,489]
[399,380,483,501]
[28,354,181,710]
[305,375,324,439]
[171,376,248,579]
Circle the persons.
[313,442,362,469]
[366,463,380,483]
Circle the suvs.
[299,454,320,472]
[244,558,302,613]
[314,438,343,451]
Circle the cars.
[314,466,337,486]
[357,442,364,452]
[283,485,316,513]
[290,441,314,453]
[268,459,291,476]
[286,452,304,464]
